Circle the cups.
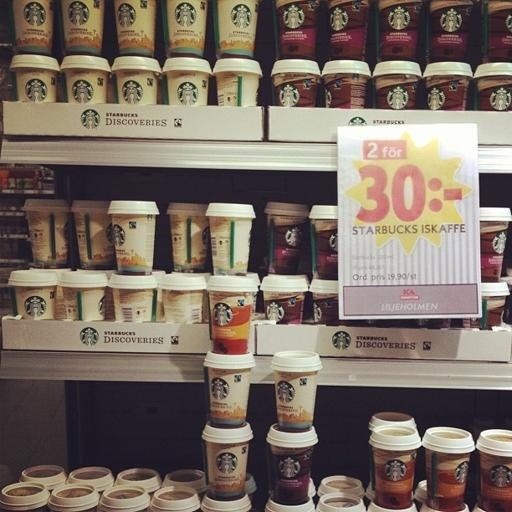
[13,1,511,112]
[0,350,512,510]
[2,199,512,357]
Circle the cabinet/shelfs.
[0,99,512,511]
